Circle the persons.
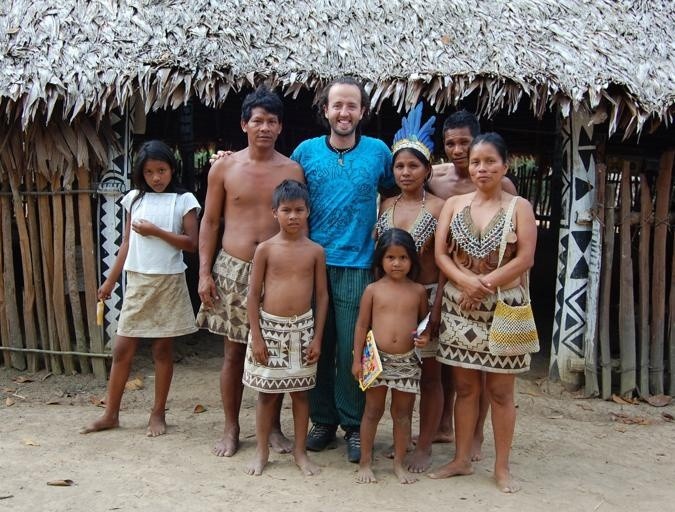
[427,110,517,462]
[208,76,401,462]
[195,89,311,458]
[371,102,447,473]
[78,140,202,438]
[352,228,430,485]
[433,131,537,494]
[242,178,330,478]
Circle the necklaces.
[330,143,354,167]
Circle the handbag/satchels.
[489,298,541,357]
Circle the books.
[352,330,383,393]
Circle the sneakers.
[345,428,361,463]
[305,421,338,452]
